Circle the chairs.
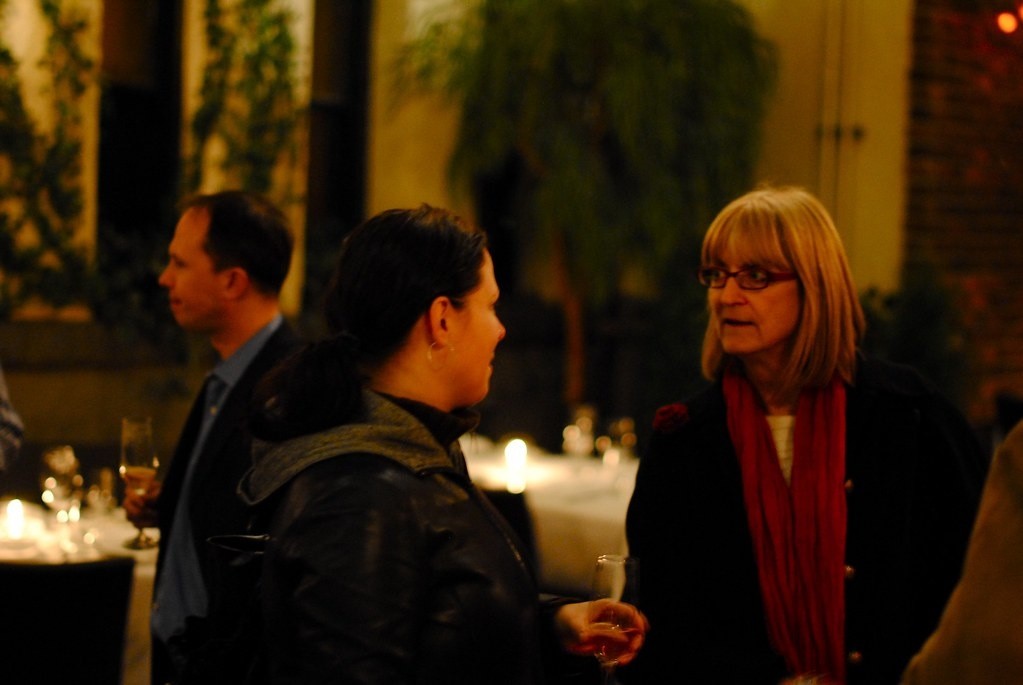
[0,555,136,685]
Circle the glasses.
[698,264,797,290]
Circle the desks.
[0,502,159,685]
[468,446,638,593]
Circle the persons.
[236,206,647,685]
[116,190,305,685]
[624,184,986,685]
[900,413,1023,685]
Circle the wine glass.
[119,415,161,550]
[583,555,647,685]
